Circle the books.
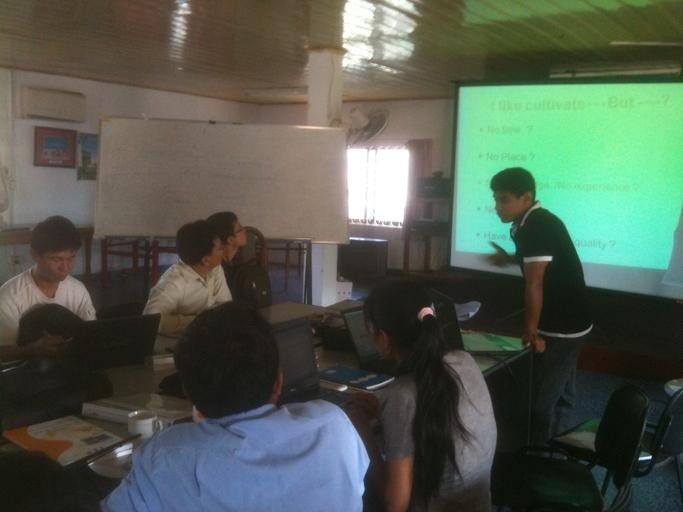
[319,365,397,390]
[460,330,523,354]
[3,416,122,466]
[83,394,194,426]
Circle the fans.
[342,107,391,148]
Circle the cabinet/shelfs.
[403,172,453,283]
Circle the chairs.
[98,302,145,321]
[495,384,651,512]
[546,387,683,512]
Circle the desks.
[0,299,537,512]
[0,226,93,278]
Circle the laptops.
[271,313,347,414]
[71,311,162,369]
[342,309,421,375]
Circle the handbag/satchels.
[221,259,272,308]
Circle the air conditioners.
[20,85,86,124]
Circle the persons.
[142,218,233,336]
[485,167,594,445]
[0,215,98,359]
[208,213,248,291]
[99,302,371,512]
[366,281,498,511]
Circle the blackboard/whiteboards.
[92,114,349,244]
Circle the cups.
[127,408,163,440]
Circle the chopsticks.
[71,432,142,470]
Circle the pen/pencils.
[42,330,50,337]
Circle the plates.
[86,442,132,481]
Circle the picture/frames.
[34,125,76,168]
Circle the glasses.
[233,224,243,235]
